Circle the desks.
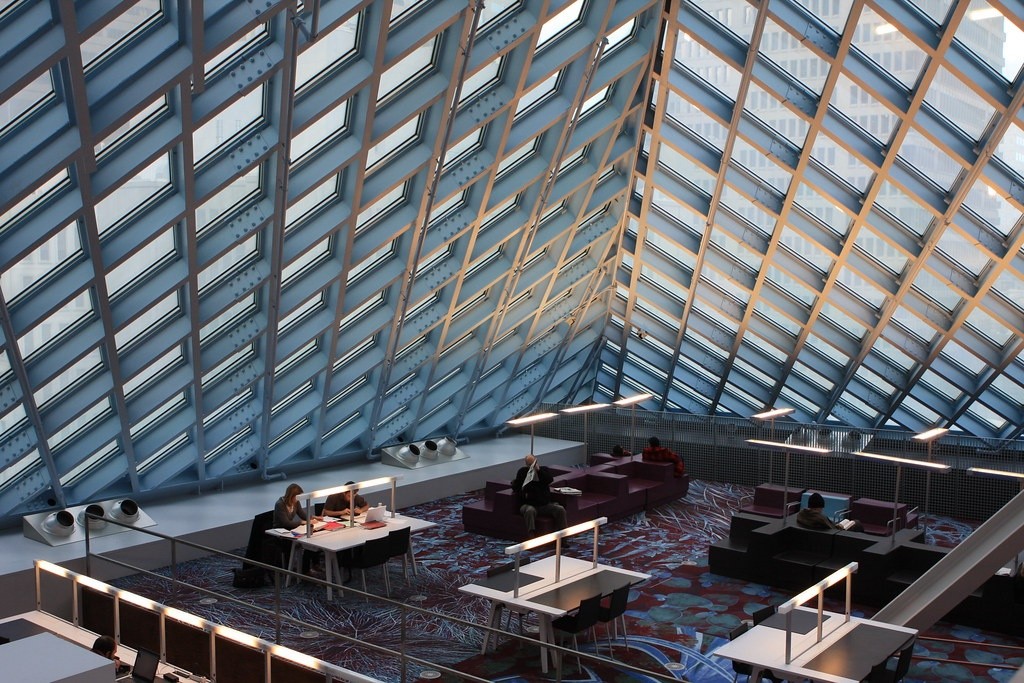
[458,554,653,674]
[265,506,438,600]
[712,606,919,683]
[0,632,117,683]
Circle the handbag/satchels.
[234,569,266,589]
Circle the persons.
[642,436,685,473]
[322,481,369,515]
[797,493,864,533]
[515,454,571,551]
[613,444,631,456]
[89,636,121,669]
[273,483,324,583]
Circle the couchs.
[707,482,1023,636]
[461,449,688,546]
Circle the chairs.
[488,556,533,648]
[343,534,393,604]
[868,657,891,683]
[886,641,915,683]
[255,511,290,580]
[729,622,780,683]
[589,581,631,660]
[551,592,604,673]
[753,605,775,627]
[387,525,411,596]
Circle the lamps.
[505,517,607,554]
[612,394,653,407]
[743,408,1024,483]
[506,412,559,427]
[559,402,613,415]
[778,562,858,614]
[295,474,404,500]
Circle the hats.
[808,493,825,508]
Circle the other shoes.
[301,564,326,582]
[554,541,569,548]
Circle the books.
[835,519,856,530]
[554,487,582,494]
[290,521,329,535]
[323,522,346,532]
[360,520,387,529]
